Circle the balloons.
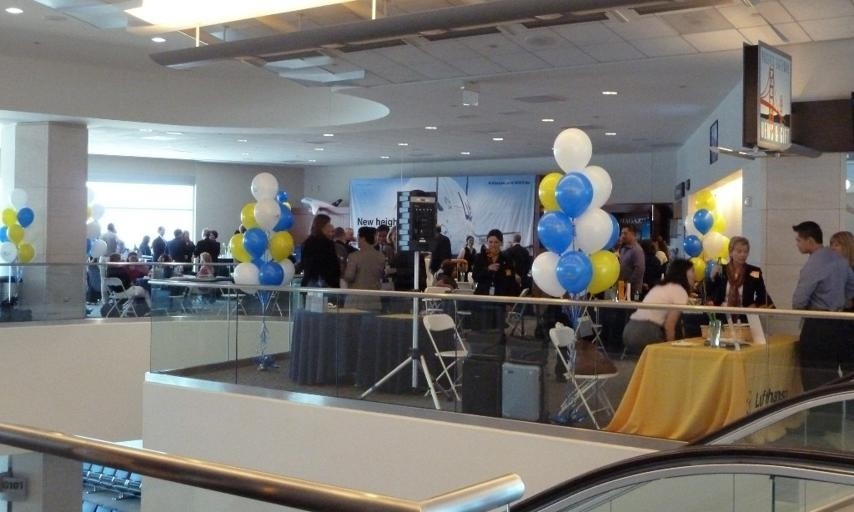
[0,189,35,263]
[229,172,295,296]
[531,131,621,298]
[87,187,107,258]
[684,187,732,281]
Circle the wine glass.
[460,272,466,283]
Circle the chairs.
[80,460,142,512]
[98,254,285,319]
[423,282,621,430]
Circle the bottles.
[633,288,641,302]
[715,257,724,283]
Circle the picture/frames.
[707,117,719,164]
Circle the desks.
[149,279,854,320]
[290,308,455,394]
[643,332,808,447]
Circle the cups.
[610,287,618,302]
[472,281,478,291]
